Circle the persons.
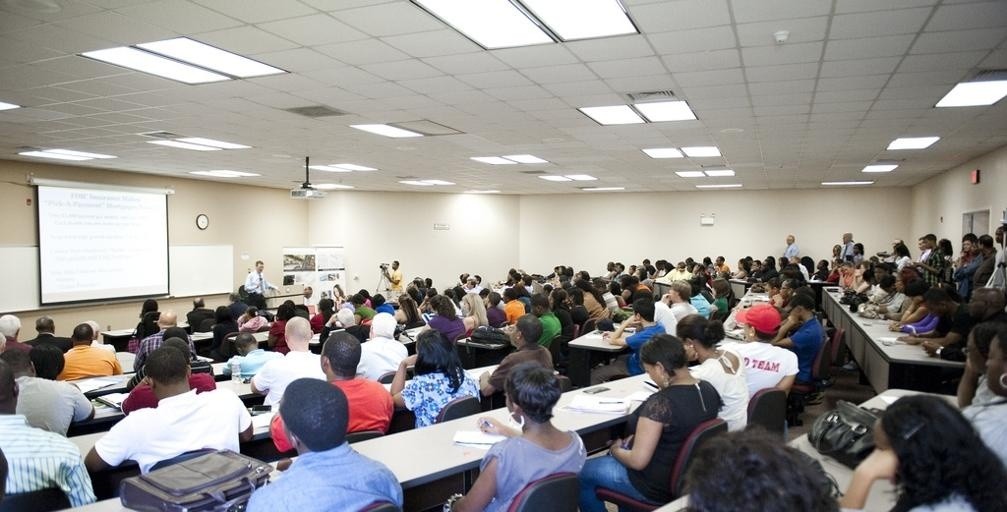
[0,231,1007,511]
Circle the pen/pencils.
[598,401,624,404]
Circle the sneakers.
[843,360,858,369]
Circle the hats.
[736,304,782,334]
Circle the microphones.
[260,278,263,290]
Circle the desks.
[54,279,965,512]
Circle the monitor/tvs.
[283,275,295,285]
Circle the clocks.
[196,214,209,230]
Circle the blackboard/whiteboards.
[0,243,234,315]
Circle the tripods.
[376,270,391,295]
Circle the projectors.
[290,190,328,198]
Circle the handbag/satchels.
[119,449,275,512]
[839,290,880,319]
[807,400,886,469]
[607,306,629,322]
[471,326,510,344]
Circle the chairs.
[0,487,72,512]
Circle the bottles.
[230,355,242,383]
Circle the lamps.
[700,211,716,225]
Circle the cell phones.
[584,387,610,394]
